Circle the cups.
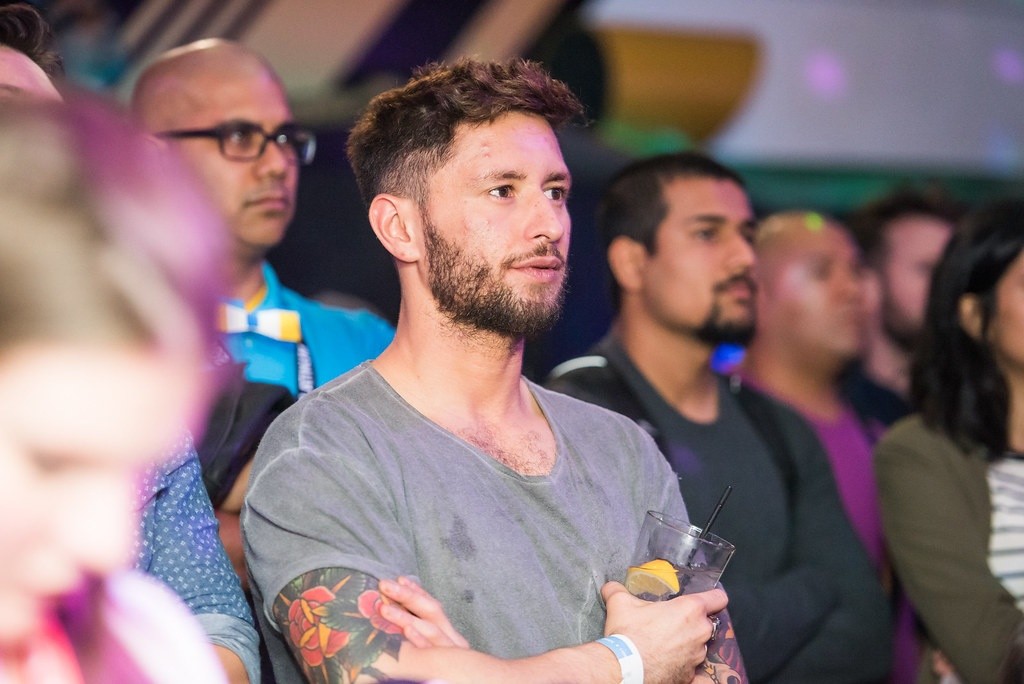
[623,510,734,602]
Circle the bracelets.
[596,634,645,683]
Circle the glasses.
[154,118,318,163]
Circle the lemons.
[626,559,680,597]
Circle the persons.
[0,0,1024,683]
